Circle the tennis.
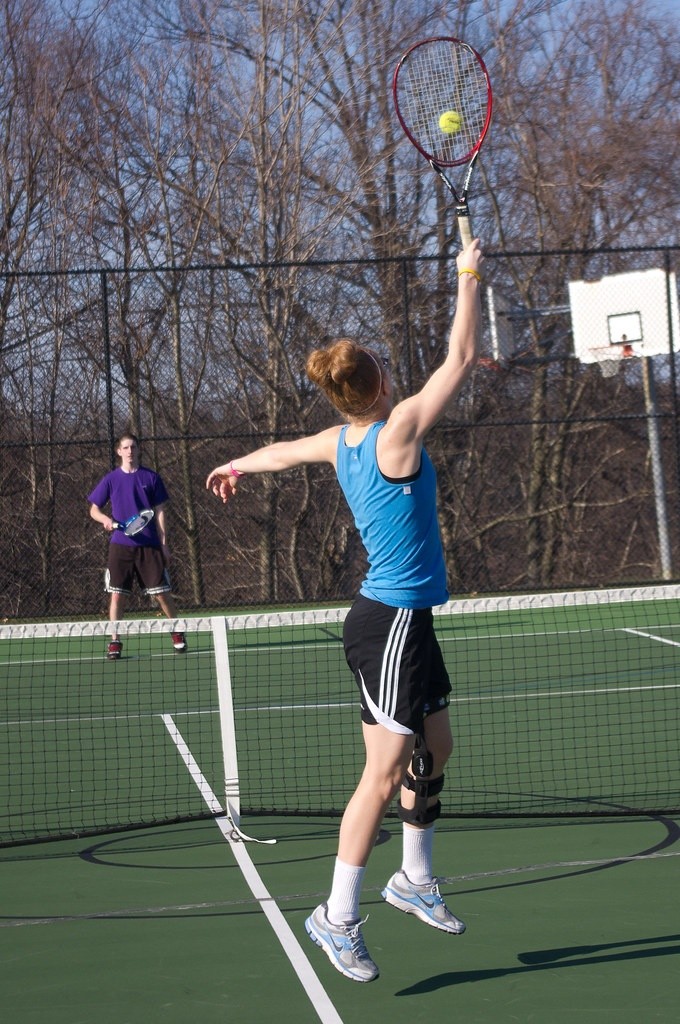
[438,110,462,136]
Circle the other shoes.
[170,632,187,652]
[108,641,122,660]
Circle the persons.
[206,237,490,981]
[88,434,188,659]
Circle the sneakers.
[382,870,466,935]
[305,902,380,983]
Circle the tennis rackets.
[112,509,154,538]
[393,33,495,253]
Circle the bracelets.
[228,459,246,479]
[458,269,481,283]
